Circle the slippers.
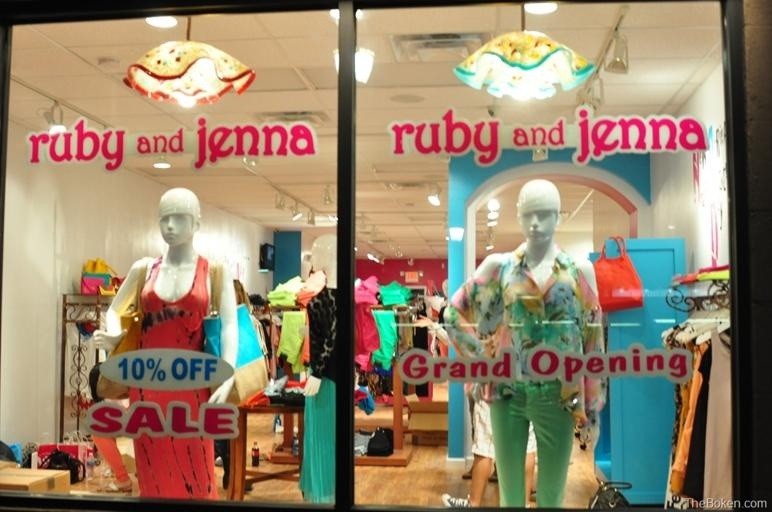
[97,481,133,493]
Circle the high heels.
[488,462,500,483]
[462,466,474,479]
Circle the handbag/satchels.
[38,449,87,484]
[111,276,125,292]
[36,430,95,481]
[367,426,393,457]
[587,476,633,510]
[97,283,117,297]
[594,235,644,314]
[80,257,117,296]
[96,309,155,400]
[201,303,270,409]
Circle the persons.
[443,179,605,506]
[93,186,239,499]
[302,234,338,504]
[442,383,537,509]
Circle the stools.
[228,403,303,502]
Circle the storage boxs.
[0,468,71,494]
[408,401,449,446]
[0,459,16,470]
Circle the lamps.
[145,16,177,29]
[428,190,442,207]
[49,102,66,136]
[153,153,171,170]
[123,16,255,109]
[453,3,596,101]
[575,6,632,116]
[482,198,501,250]
[271,181,338,228]
[521,3,559,15]
[332,47,376,86]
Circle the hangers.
[661,316,731,347]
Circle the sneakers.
[441,493,472,509]
[222,474,252,491]
